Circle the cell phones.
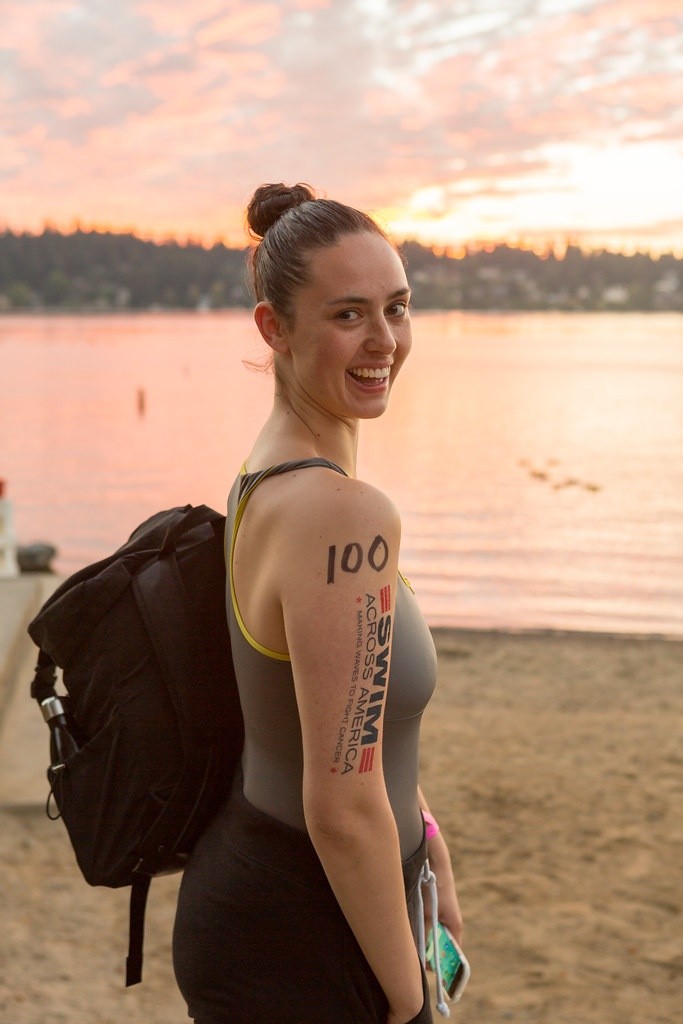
[426,922,471,1006]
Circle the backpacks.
[27,503,245,891]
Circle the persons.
[173,183,464,1024]
